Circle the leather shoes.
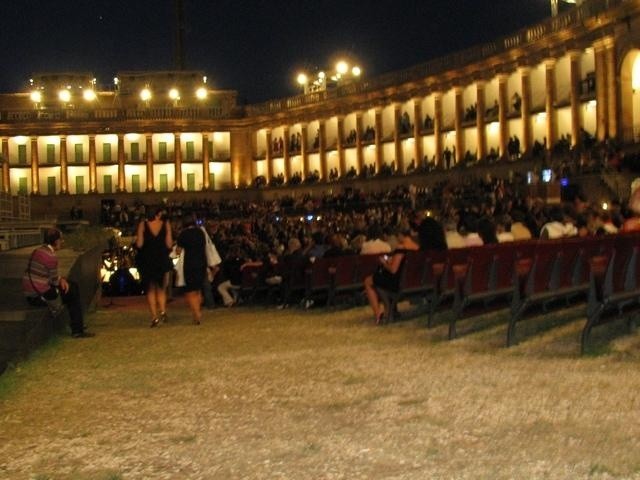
[71,331,96,339]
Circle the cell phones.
[383,253,388,261]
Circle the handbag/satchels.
[153,248,172,272]
[204,243,222,267]
[371,265,384,288]
[46,294,68,318]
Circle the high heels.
[150,312,170,328]
[374,311,384,326]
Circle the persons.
[22,227,95,339]
[134,206,173,327]
[176,214,219,325]
[0,94,640,312]
[364,217,420,323]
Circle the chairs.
[211,232,640,354]
